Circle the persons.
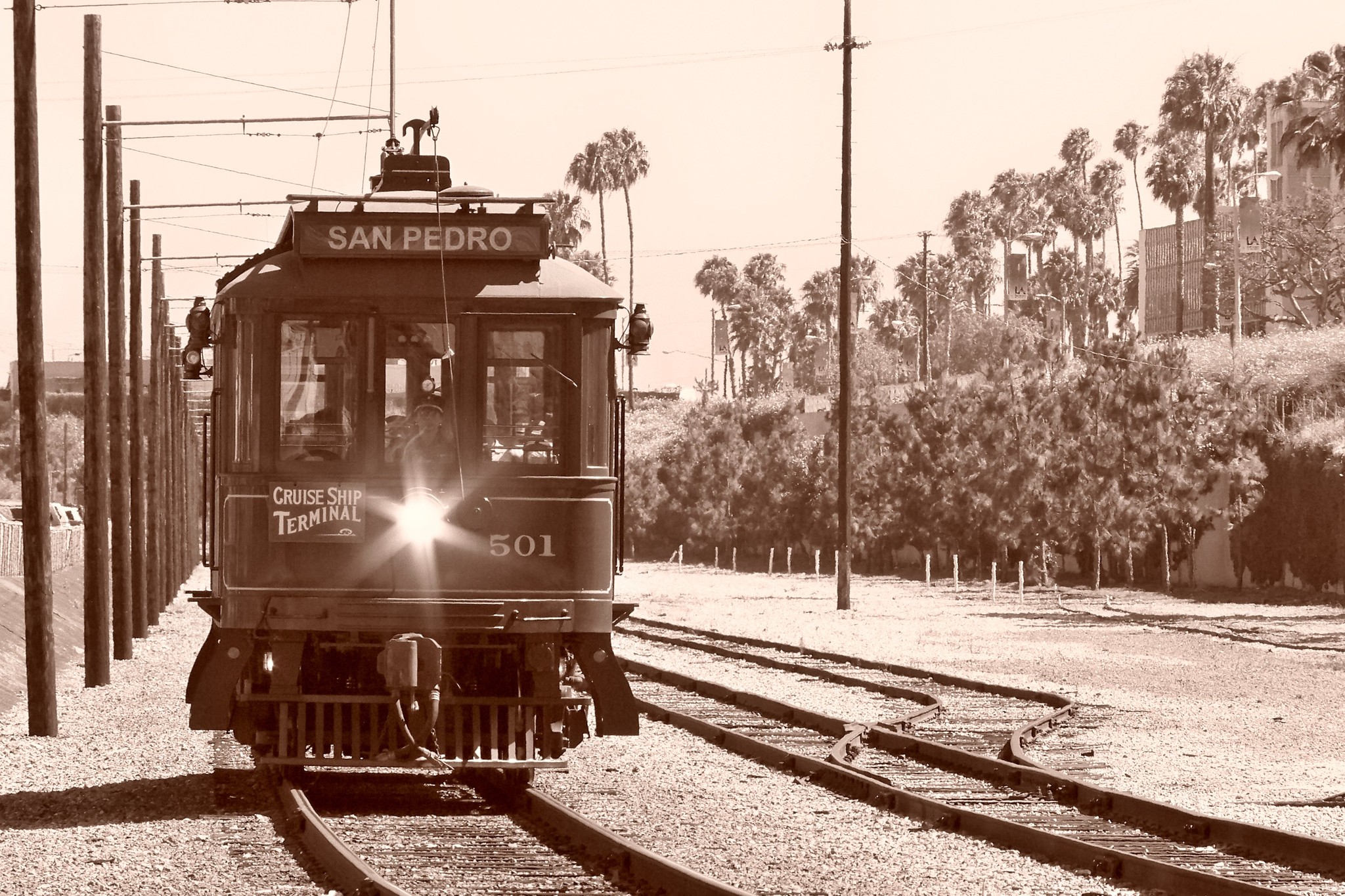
[384,394,457,466]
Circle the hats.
[384,414,406,431]
[413,393,443,413]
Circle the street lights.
[1230,171,1282,336]
[710,303,742,398]
[1001,232,1047,367]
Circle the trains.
[177,104,654,802]
